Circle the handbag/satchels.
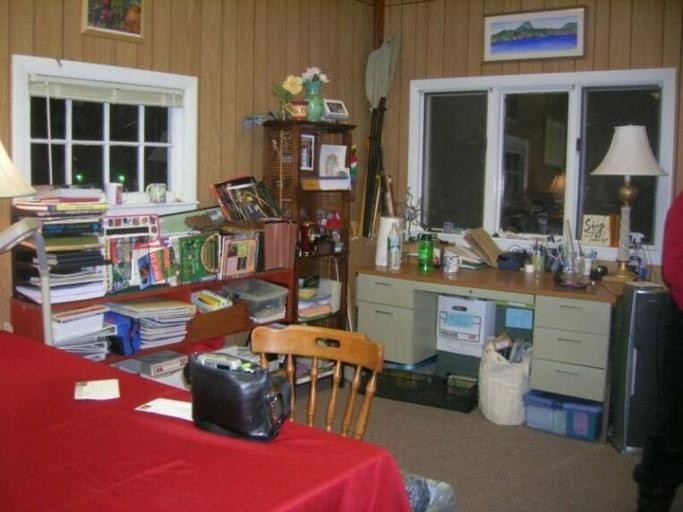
[188,350,284,442]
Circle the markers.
[199,293,229,307]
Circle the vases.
[304,81,327,122]
[283,98,314,123]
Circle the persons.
[632,192,683,512]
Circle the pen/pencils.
[535,240,545,256]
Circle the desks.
[1,327,414,512]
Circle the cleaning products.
[626,231,648,280]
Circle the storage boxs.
[219,277,288,319]
[519,389,606,442]
[357,349,485,416]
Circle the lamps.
[586,122,669,285]
[0,134,41,205]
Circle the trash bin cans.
[399,470,454,512]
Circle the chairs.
[249,321,386,444]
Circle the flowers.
[280,73,307,97]
[302,65,331,86]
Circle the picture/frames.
[299,132,316,171]
[322,98,351,117]
[479,2,590,65]
[78,0,148,45]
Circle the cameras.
[197,352,241,371]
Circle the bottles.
[301,221,312,257]
[385,222,400,275]
[581,253,607,280]
[431,239,440,267]
[415,231,435,274]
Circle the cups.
[442,253,461,274]
[519,254,544,272]
[104,182,128,206]
[146,182,166,204]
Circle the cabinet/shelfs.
[8,220,295,391]
[264,118,355,387]
[357,260,629,427]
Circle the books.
[11,196,197,362]
